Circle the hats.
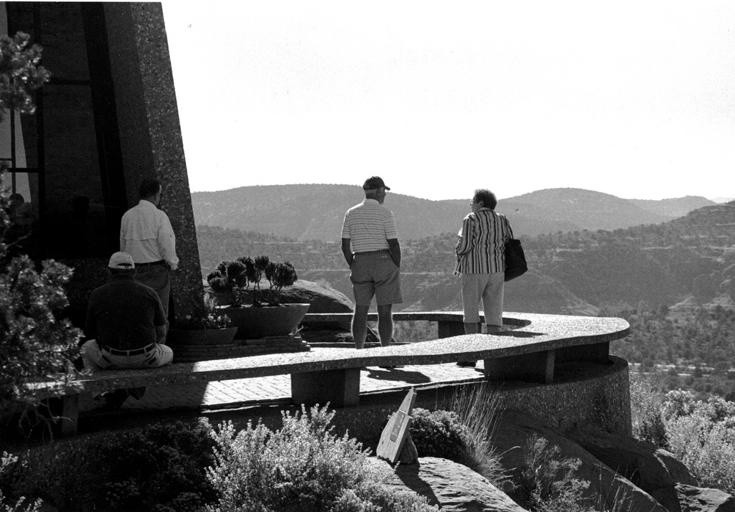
[108,252,136,270]
[363,176,390,191]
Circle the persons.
[1,191,27,247]
[451,188,514,369]
[117,177,184,347]
[74,250,178,375]
[339,175,406,369]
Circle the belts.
[104,344,155,356]
[135,259,165,267]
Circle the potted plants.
[201,303,237,343]
[208,255,311,337]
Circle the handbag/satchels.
[504,217,528,282]
